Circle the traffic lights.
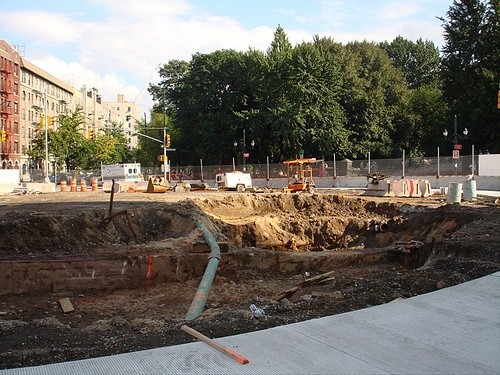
[166,133,170,146]
[2,130,6,142]
[88,129,93,140]
[48,115,54,126]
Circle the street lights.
[125,111,147,181]
[233,129,255,173]
[442,114,468,176]
